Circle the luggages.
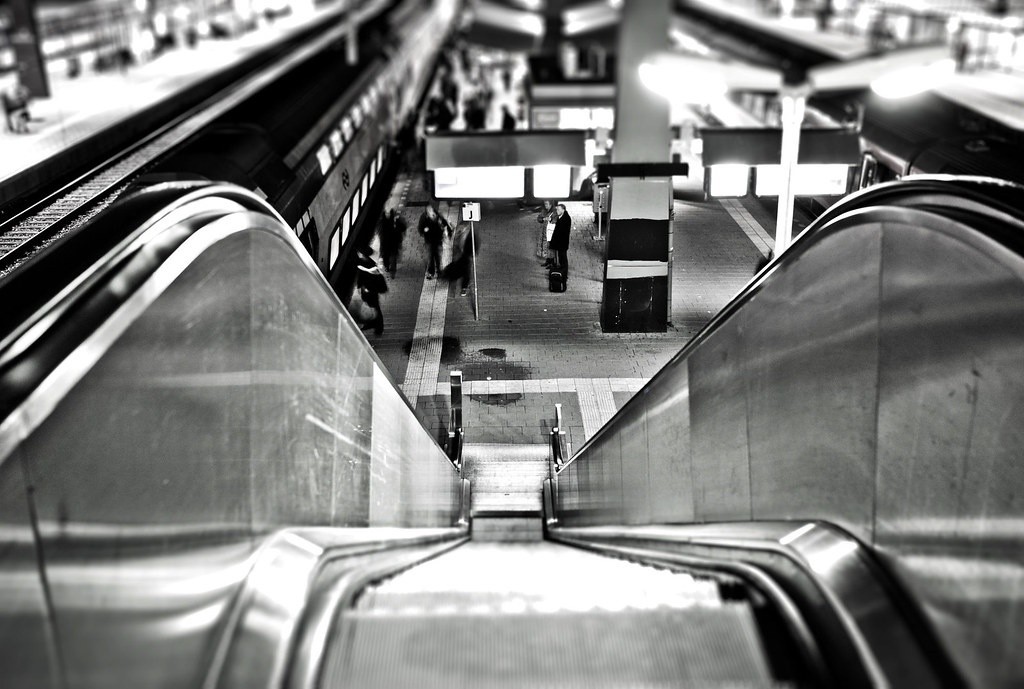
[548,265,567,293]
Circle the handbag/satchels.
[546,212,557,241]
[360,301,376,320]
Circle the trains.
[0,0,459,409]
[668,0,1024,207]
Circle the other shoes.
[361,324,373,331]
[541,262,549,267]
[385,267,389,272]
[460,289,466,296]
[389,268,397,280]
[426,272,434,279]
[374,329,382,339]
[546,264,553,269]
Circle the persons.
[536,199,571,293]
[417,204,446,279]
[356,242,388,336]
[376,199,407,279]
[444,221,478,298]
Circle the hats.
[356,243,374,258]
